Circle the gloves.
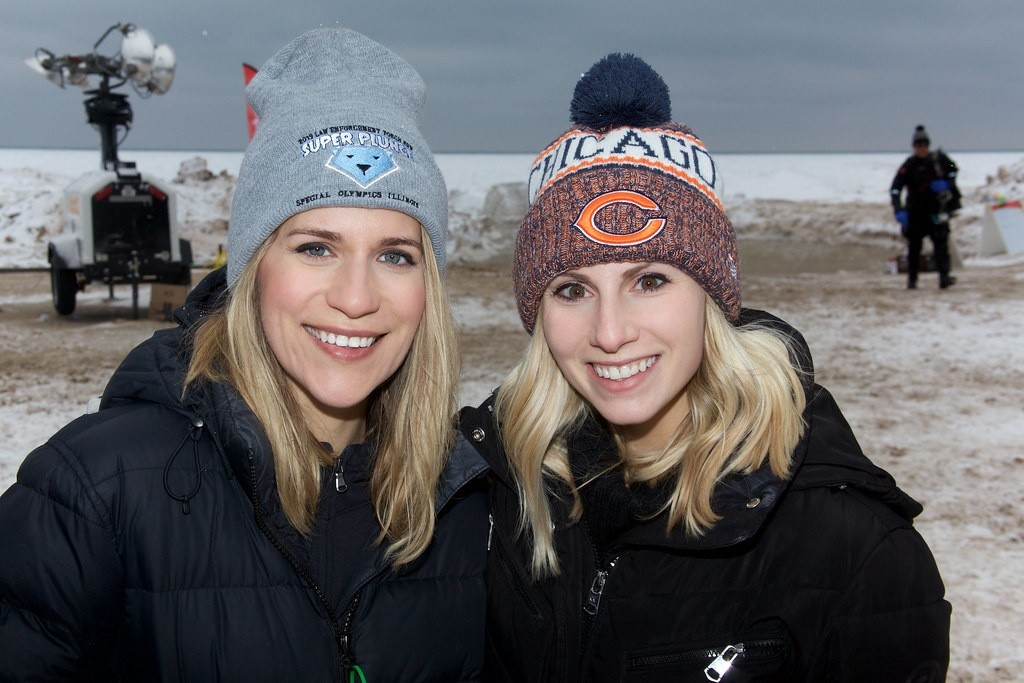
[895,210,907,224]
[933,179,948,192]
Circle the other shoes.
[908,272,918,288]
[937,268,957,289]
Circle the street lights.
[22,19,177,167]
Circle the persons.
[456,120,954,676]
[0,88,512,682]
[891,123,963,284]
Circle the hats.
[223,30,447,286]
[910,125,931,152]
[515,52,743,333]
